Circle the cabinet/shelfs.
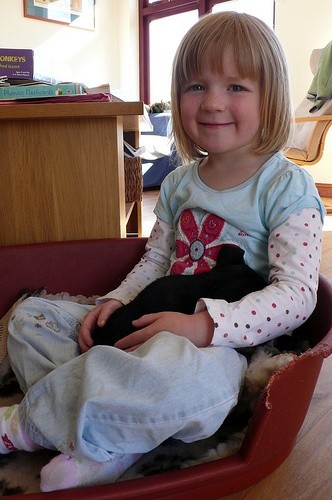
[0,102,144,247]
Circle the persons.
[0,11,327,491]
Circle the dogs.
[78,244,280,363]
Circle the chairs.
[281,40,332,213]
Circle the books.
[0,83,88,99]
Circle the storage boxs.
[0,49,54,84]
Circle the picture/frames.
[24,0,96,31]
